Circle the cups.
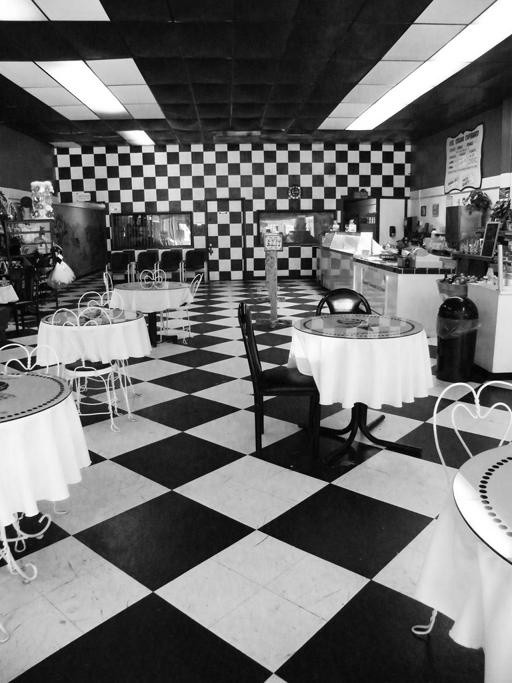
[362,249,369,259]
[396,257,410,266]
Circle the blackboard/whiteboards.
[480,222,501,259]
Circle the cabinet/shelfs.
[466,278,512,374]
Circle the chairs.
[236,300,322,453]
[101,247,210,348]
[76,290,143,405]
[9,266,42,335]
[49,305,137,434]
[0,342,60,379]
[409,378,512,636]
[313,287,374,317]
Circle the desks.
[284,311,435,466]
[0,370,93,585]
[34,305,155,419]
[416,444,512,683]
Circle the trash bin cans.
[436,295,482,383]
